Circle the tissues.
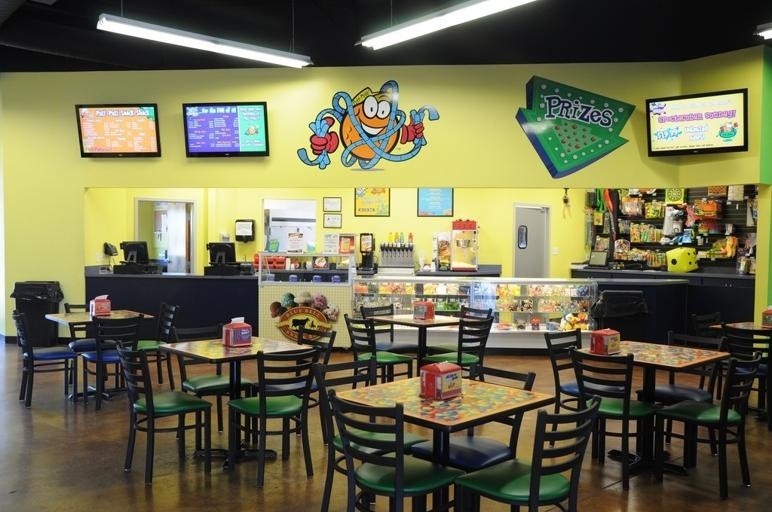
[761,305,772,328]
[413,300,435,320]
[222,317,253,346]
[89,294,111,316]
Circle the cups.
[267,274,275,282]
[332,275,341,282]
[531,319,539,330]
[330,263,336,269]
[290,275,298,281]
[313,274,323,282]
[286,258,313,270]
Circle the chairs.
[410,363,536,512]
[44,303,156,411]
[12,310,78,408]
[344,313,412,389]
[454,395,602,512]
[636,330,728,455]
[312,355,430,512]
[359,301,494,387]
[226,345,323,488]
[117,340,213,488]
[655,351,762,500]
[544,328,625,459]
[136,302,179,390]
[572,352,662,491]
[171,323,256,450]
[252,324,336,450]
[707,310,772,432]
[328,389,467,512]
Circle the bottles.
[387,231,416,244]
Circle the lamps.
[351,0,540,53]
[95,1,315,71]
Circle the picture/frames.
[323,212,342,229]
[417,187,454,217]
[323,197,343,213]
[354,187,391,217]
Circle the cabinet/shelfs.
[569,184,759,361]
[350,274,599,359]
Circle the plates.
[315,257,326,267]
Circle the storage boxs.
[89,299,111,316]
[223,323,252,347]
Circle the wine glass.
[419,250,426,272]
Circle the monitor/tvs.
[123,241,149,261]
[208,242,237,261]
[180,102,270,157]
[75,102,161,159]
[643,87,749,156]
